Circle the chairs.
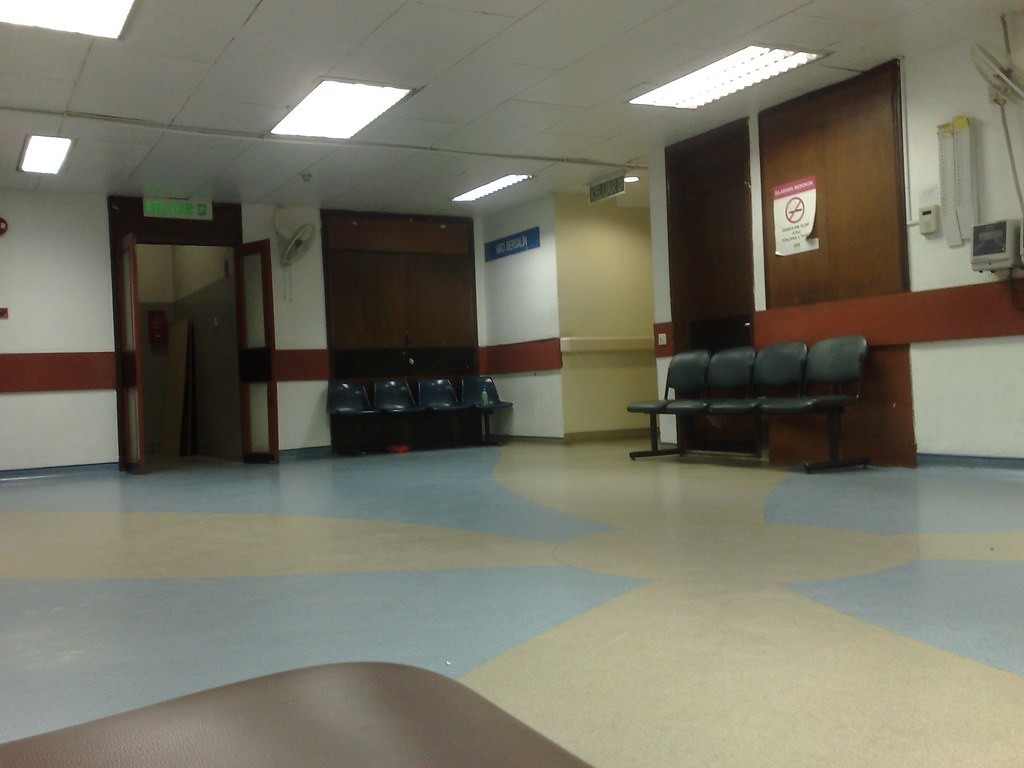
[626,334,871,474]
[325,376,514,457]
[0,662,591,768]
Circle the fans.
[274,223,317,267]
